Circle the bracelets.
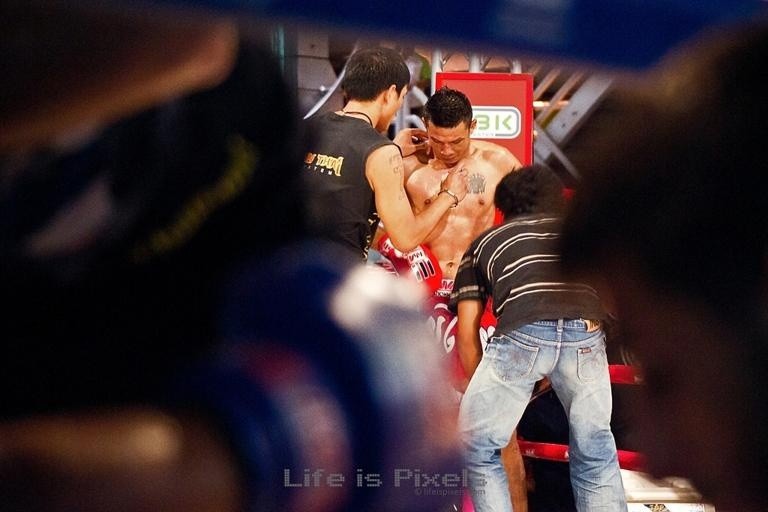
[436,188,459,209]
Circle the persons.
[370,83,529,512]
[559,23,767,512]
[446,160,629,512]
[300,44,470,270]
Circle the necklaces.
[340,108,374,128]
